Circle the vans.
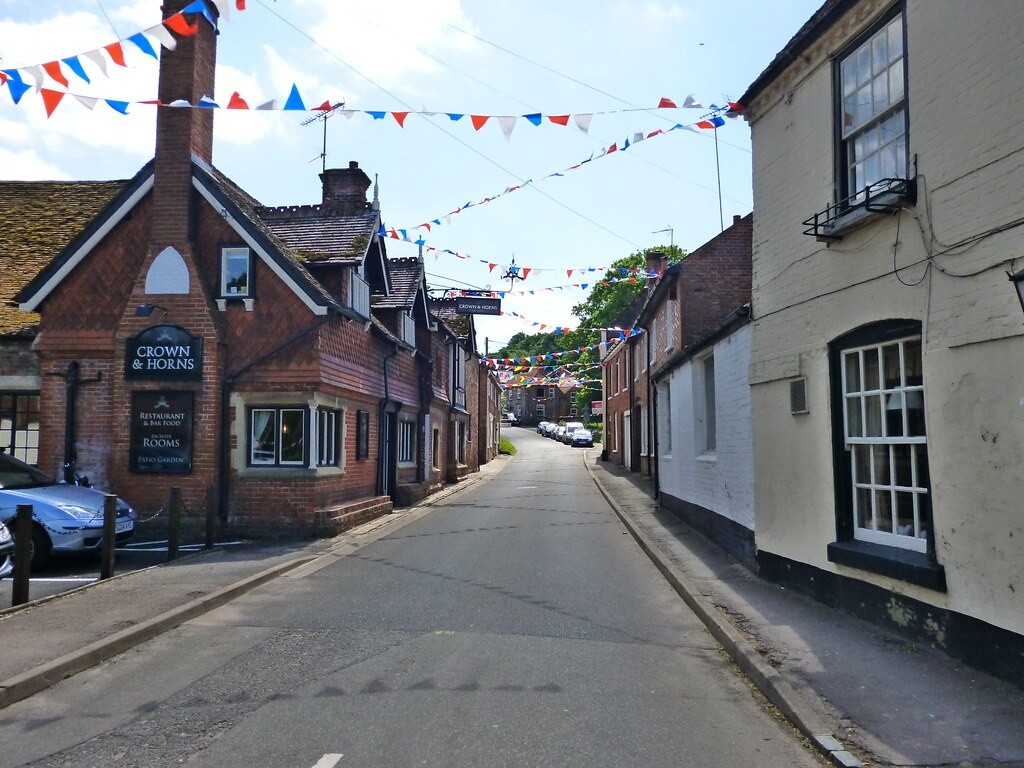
[563,422,585,445]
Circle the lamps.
[134,303,156,317]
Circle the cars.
[555,427,565,442]
[571,429,594,447]
[0,521,16,580]
[537,422,549,434]
[550,426,559,439]
[506,413,522,427]
[542,424,558,437]
[0,452,136,573]
